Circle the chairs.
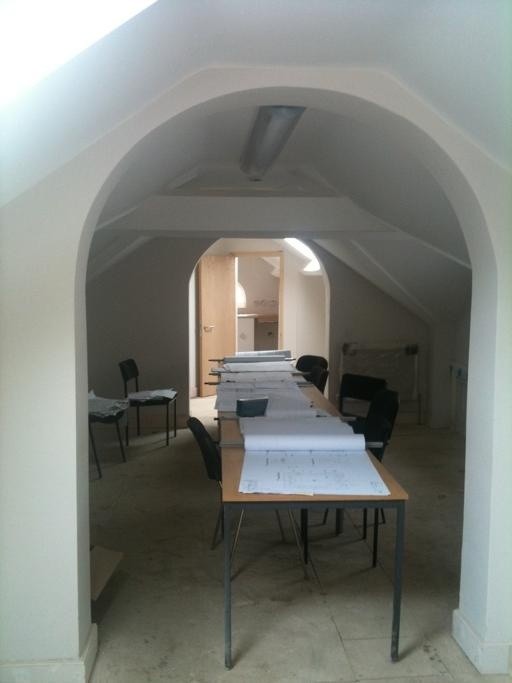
[296,355,398,567]
[119,359,179,446]
[89,411,127,478]
[187,418,285,549]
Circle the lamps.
[236,106,306,180]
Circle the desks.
[217,361,408,668]
